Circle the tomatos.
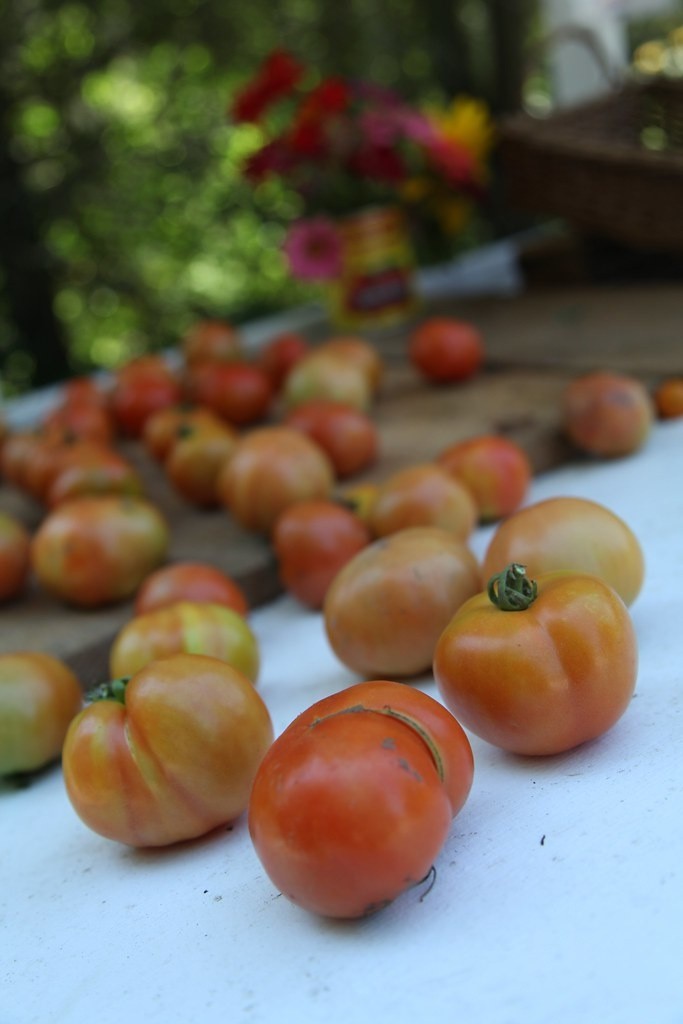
[1,319,680,927]
